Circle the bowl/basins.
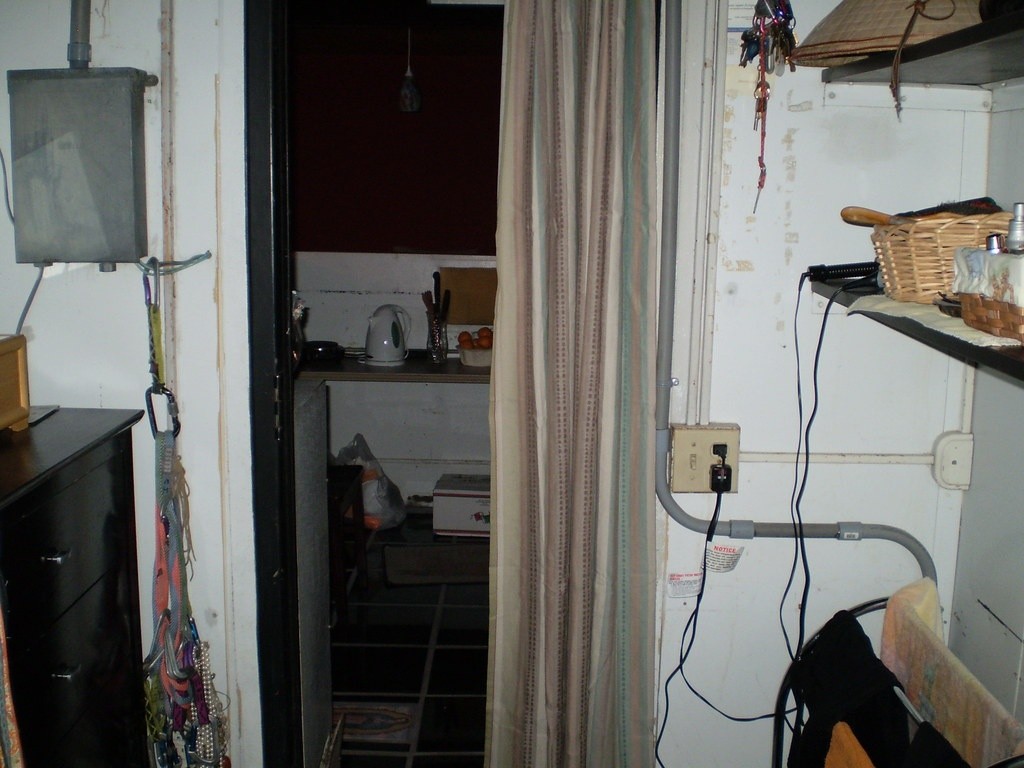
[302,341,345,370]
[456,344,492,367]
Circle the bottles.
[427,320,449,364]
[362,471,383,528]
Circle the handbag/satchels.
[335,433,406,529]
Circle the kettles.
[365,304,412,367]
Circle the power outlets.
[668,424,741,493]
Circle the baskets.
[871,212,1015,304]
[950,245,1024,342]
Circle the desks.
[328,465,370,633]
[296,349,494,383]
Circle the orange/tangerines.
[458,327,493,350]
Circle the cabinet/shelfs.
[810,10,1024,381]
[0,404,149,768]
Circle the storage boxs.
[430,472,492,539]
[437,267,496,358]
